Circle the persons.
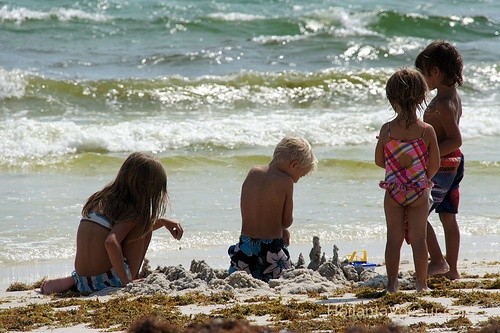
[228,135,316,281]
[415,40,465,280]
[41,152,184,296]
[374,67,441,295]
